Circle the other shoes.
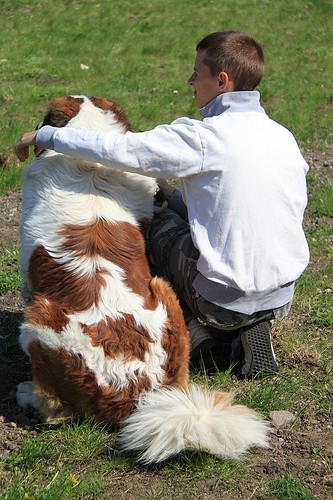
[186,318,215,373]
[238,323,280,380]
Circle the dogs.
[14,94,279,464]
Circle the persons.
[15,31,311,375]
